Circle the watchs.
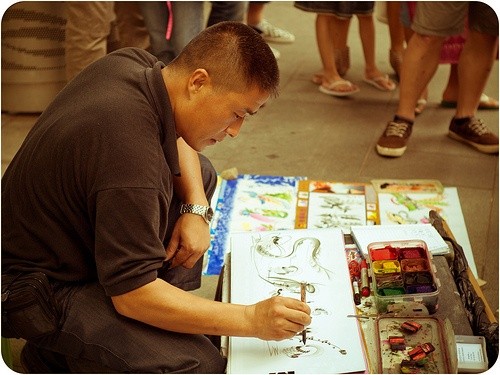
[179,203,214,225]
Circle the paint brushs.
[301,283,307,345]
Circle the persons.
[65,2,499,157]
[1,21,310,373]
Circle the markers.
[352,260,370,305]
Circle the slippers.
[318,80,361,97]
[362,73,396,91]
[441,92,499,110]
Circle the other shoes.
[19,344,72,374]
[447,116,499,154]
[311,62,347,85]
[375,119,413,157]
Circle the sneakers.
[248,20,295,44]
